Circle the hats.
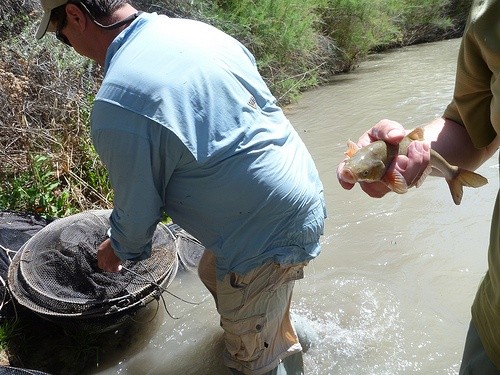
[35,0,72,40]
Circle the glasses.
[55,10,73,47]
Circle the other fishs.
[340,126,489,206]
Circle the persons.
[36,0,327,375]
[337,0,500,375]
